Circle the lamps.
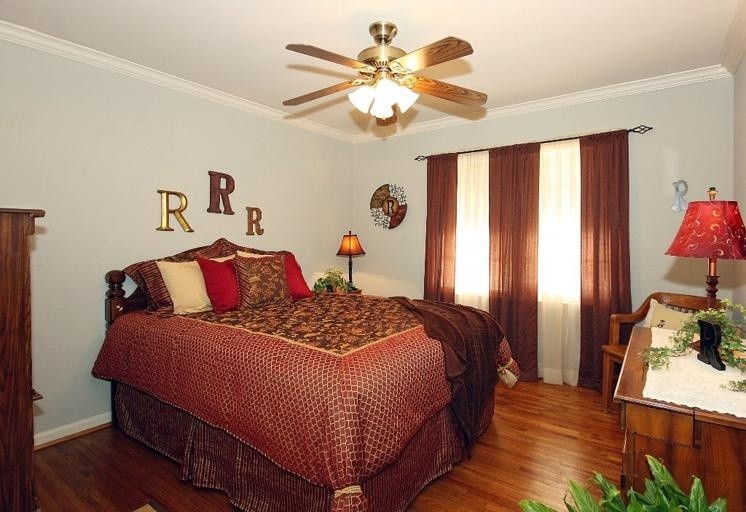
[336,231,366,296]
[350,72,417,123]
[663,186,746,299]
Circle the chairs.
[600,291,724,414]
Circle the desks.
[611,325,745,509]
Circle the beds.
[104,269,503,511]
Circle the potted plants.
[313,268,353,296]
[637,301,745,393]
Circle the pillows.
[121,238,312,318]
[635,298,694,332]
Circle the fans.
[282,19,489,127]
